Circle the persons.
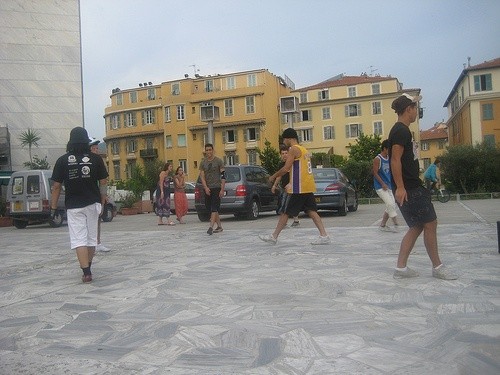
[271,147,301,229]
[198,144,225,235]
[157,163,177,226]
[388,93,459,280]
[87,136,109,251]
[372,139,404,233]
[424,156,442,192]
[174,167,188,224]
[258,128,329,244]
[49,126,111,282]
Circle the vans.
[6,169,118,228]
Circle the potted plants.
[113,163,153,216]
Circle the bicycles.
[424,181,451,203]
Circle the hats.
[69,126,92,144]
[88,136,100,145]
[391,91,422,112]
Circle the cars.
[310,168,359,217]
[152,181,198,215]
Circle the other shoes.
[207,226,213,235]
[258,233,278,245]
[311,234,330,244]
[168,221,176,224]
[82,274,92,282]
[431,263,457,279]
[213,225,223,232]
[393,266,419,279]
[378,224,391,231]
[290,219,300,227]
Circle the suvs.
[195,163,283,220]
